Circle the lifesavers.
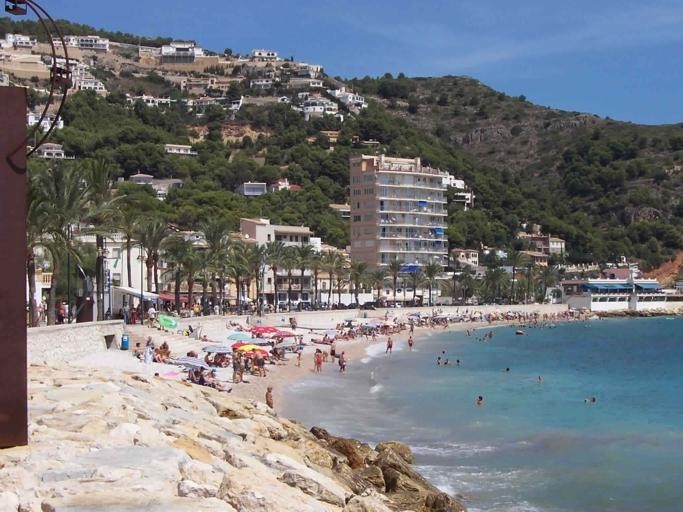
[160,315,176,328]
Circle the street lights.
[152,224,179,258]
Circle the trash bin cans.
[120,333,129,350]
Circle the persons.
[23,301,596,409]
